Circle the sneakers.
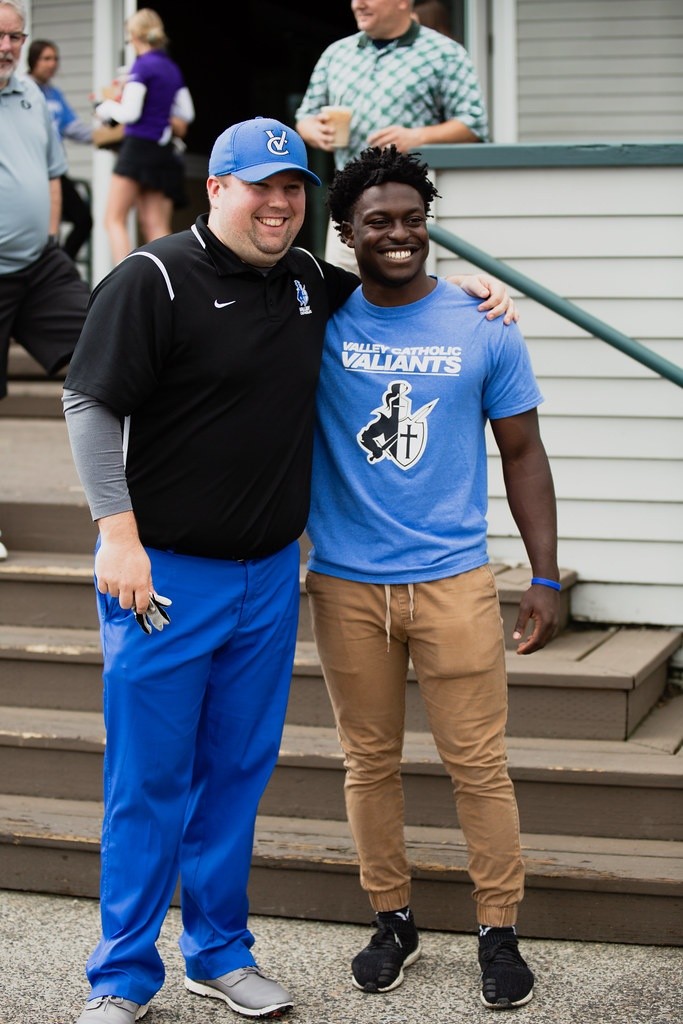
[77,995,150,1024]
[352,918,423,992]
[478,928,535,1009]
[185,966,294,1017]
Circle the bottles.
[111,80,122,102]
[88,94,119,127]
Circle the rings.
[387,144,390,148]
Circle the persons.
[296,0,489,280]
[61,117,519,1024]
[27,40,93,261]
[97,9,194,269]
[0,0,93,562]
[303,144,561,1007]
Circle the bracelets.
[531,578,560,591]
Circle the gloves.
[131,593,173,635]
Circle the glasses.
[1,29,28,47]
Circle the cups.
[320,106,353,147]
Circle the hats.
[208,116,321,187]
